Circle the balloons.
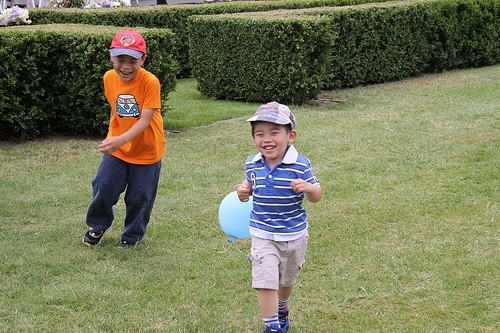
[218,190,253,243]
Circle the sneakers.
[264,305,290,333]
[81,224,112,248]
[118,237,138,248]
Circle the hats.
[246,100,295,129]
[110,29,146,58]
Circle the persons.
[237,102,322,333]
[82,29,166,249]
